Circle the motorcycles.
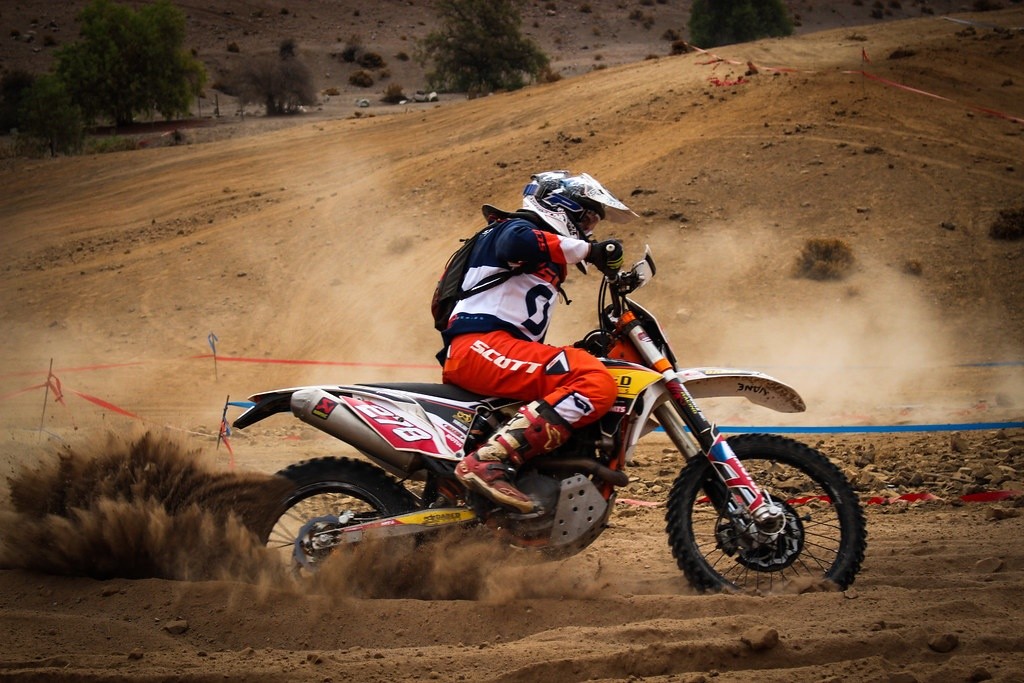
[229,244,872,594]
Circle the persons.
[442,172,636,509]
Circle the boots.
[454,401,575,514]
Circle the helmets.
[523,170,606,274]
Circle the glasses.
[577,209,601,236]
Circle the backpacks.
[431,214,548,333]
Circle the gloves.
[584,240,623,276]
